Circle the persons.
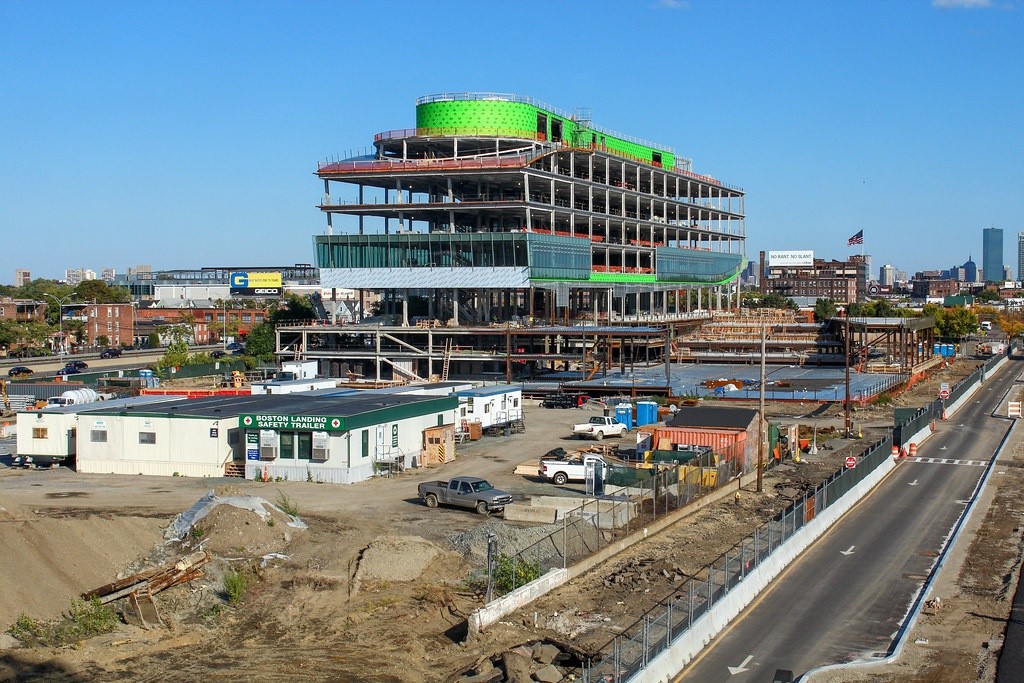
[493,315,498,323]
[735,490,741,504]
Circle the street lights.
[211,291,239,350]
[43,292,78,363]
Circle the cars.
[57,366,81,375]
[8,366,33,377]
[65,360,88,370]
[1019,332,1024,337]
[227,343,244,349]
[210,350,226,358]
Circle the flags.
[847,229,863,247]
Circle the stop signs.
[847,458,856,467]
[940,391,949,398]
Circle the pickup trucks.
[538,454,626,485]
[100,348,122,358]
[417,476,513,516]
[572,416,628,442]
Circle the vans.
[981,321,992,330]
